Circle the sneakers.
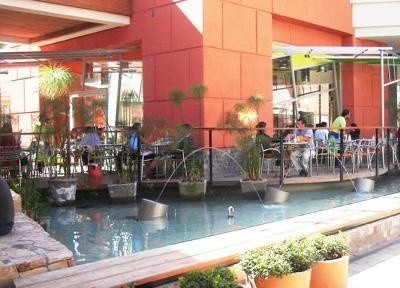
[300,168,308,177]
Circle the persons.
[135,124,193,181]
[77,126,101,172]
[390,110,400,164]
[254,109,361,177]
[128,122,163,178]
[0,175,14,236]
[0,122,29,177]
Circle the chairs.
[122,147,189,180]
[260,144,284,176]
[316,137,392,174]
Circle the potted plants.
[76,89,138,199]
[169,85,207,197]
[178,265,246,288]
[39,101,78,204]
[225,95,268,193]
[241,241,312,288]
[310,230,351,288]
